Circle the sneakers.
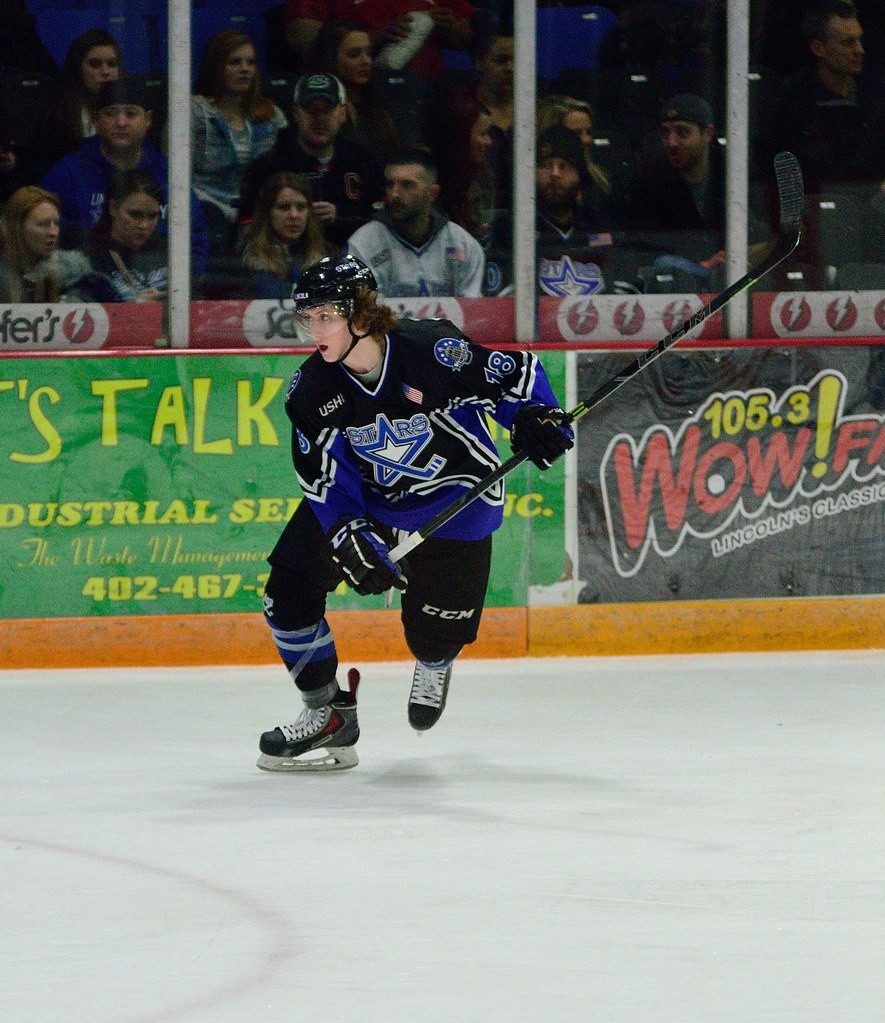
[256,668,361,772]
[408,659,452,736]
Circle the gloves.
[324,517,408,597]
[510,405,575,471]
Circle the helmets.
[293,255,378,318]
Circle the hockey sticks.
[381,148,807,562]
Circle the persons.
[256,257,575,771]
[0,0,885,306]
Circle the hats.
[294,73,346,106]
[94,81,151,111]
[659,94,713,125]
[537,126,584,163]
